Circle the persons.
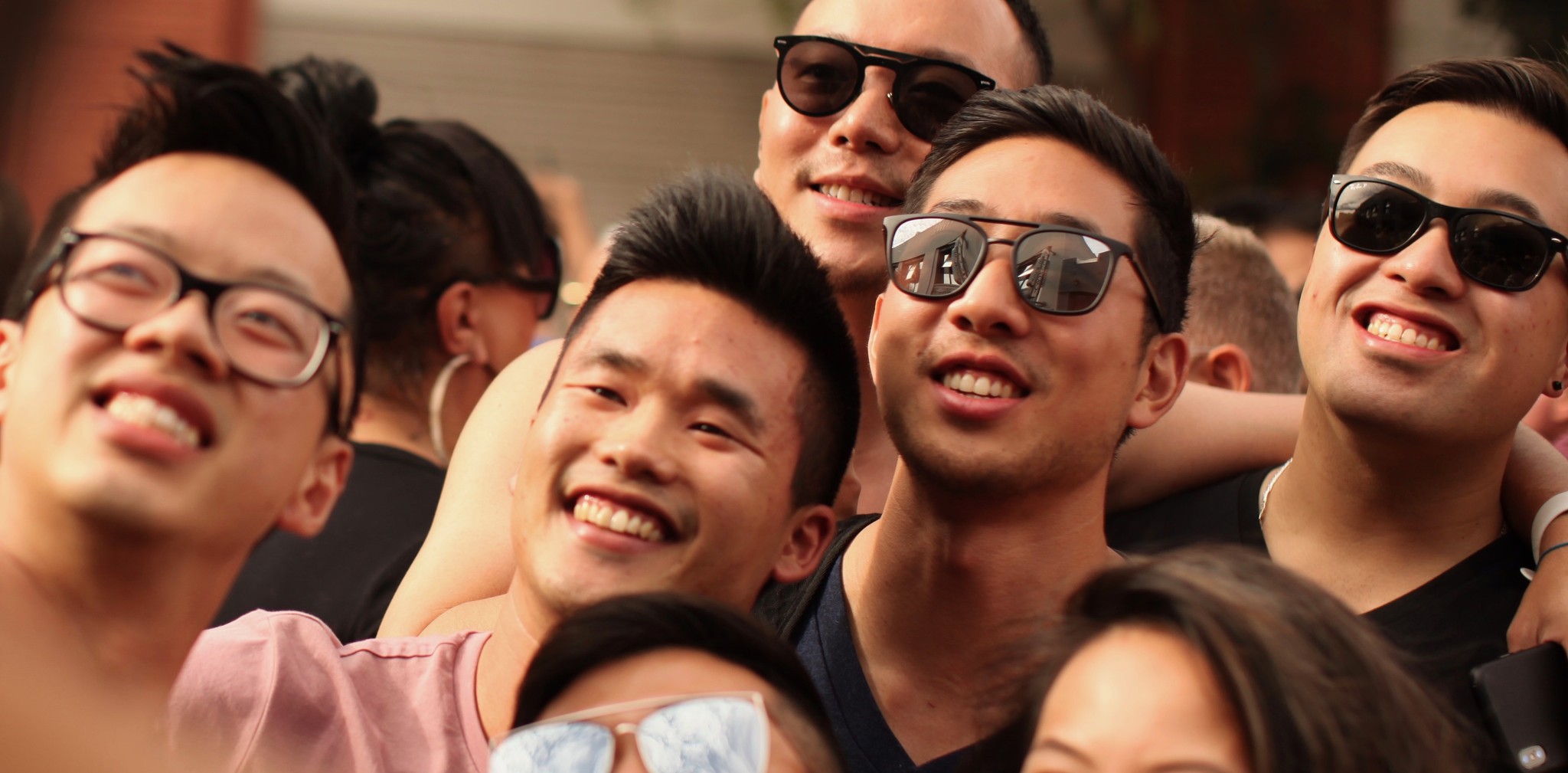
[1004,547,1493,773]
[739,83,1190,773]
[1165,189,1568,453]
[481,595,846,769]
[2,34,367,770]
[205,51,559,642]
[172,156,861,773]
[1098,55,1568,771]
[367,0,1568,653]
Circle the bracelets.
[1540,542,1567,561]
[1520,490,1568,580]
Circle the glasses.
[1328,174,1568,293]
[22,228,346,439]
[882,212,1166,335]
[418,237,565,323]
[486,690,773,773]
[773,34,997,143]
